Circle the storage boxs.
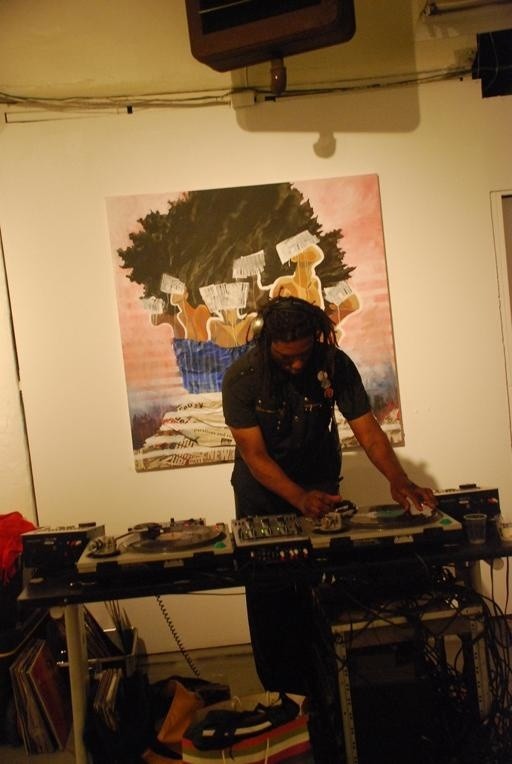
[55,623,139,698]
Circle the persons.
[222,296,440,693]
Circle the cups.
[29,576,44,594]
[464,514,488,545]
[495,513,512,542]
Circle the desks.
[17,518,512,763]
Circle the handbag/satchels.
[180,689,315,763]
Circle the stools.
[311,579,492,762]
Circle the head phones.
[250,297,323,344]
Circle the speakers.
[475,28,512,98]
